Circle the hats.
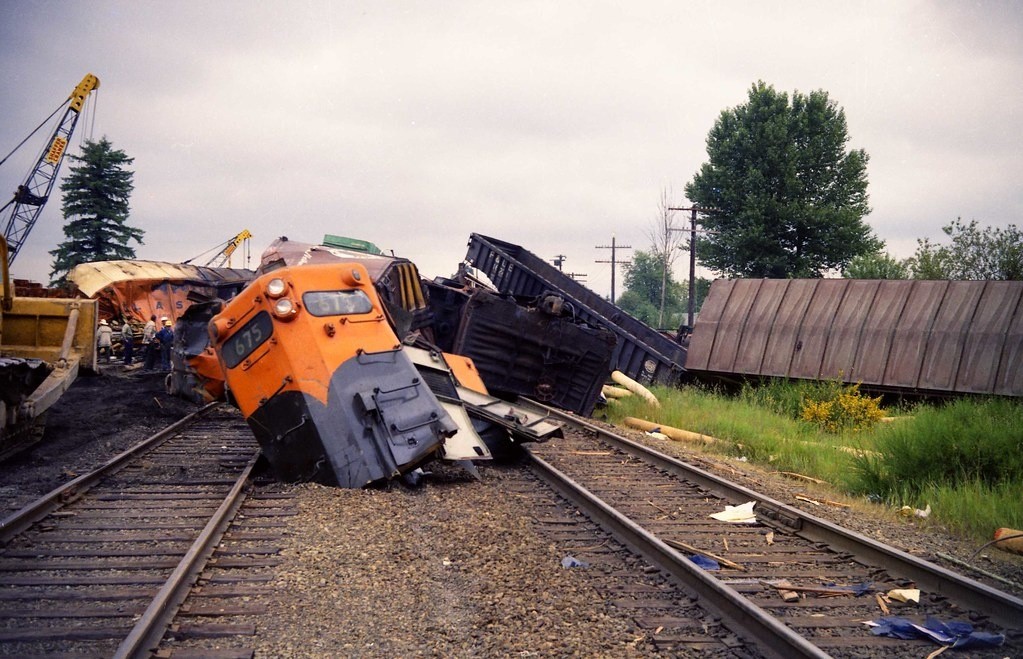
[100,319,109,325]
[161,317,168,321]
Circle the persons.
[156,317,175,371]
[122,316,134,365]
[141,315,156,372]
[96,318,112,362]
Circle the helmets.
[165,321,172,326]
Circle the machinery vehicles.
[180,229,253,271]
[1,70,103,463]
